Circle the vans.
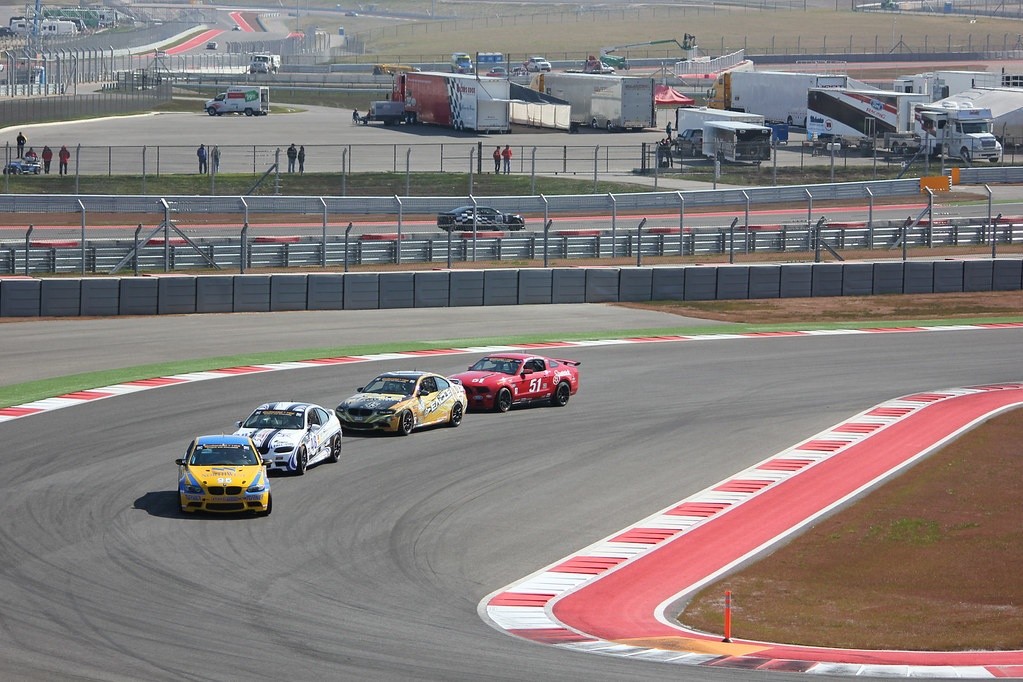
[0,27,18,37]
[451,54,474,72]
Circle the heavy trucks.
[92,10,119,28]
[386,71,512,133]
[806,86,1003,161]
[529,73,656,132]
[705,70,847,128]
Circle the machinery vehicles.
[599,34,699,70]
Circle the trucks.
[204,85,271,116]
[250,55,281,75]
[381,64,421,75]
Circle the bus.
[43,9,100,30]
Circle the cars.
[448,352,581,412]
[233,402,343,474]
[154,51,170,59]
[175,435,273,519]
[336,369,470,436]
[232,25,241,31]
[345,11,358,17]
[437,204,526,232]
[207,42,217,49]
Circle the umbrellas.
[655,86,694,125]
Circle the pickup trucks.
[486,67,529,76]
[565,56,615,74]
[674,129,704,156]
[524,57,551,73]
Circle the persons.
[656,138,673,168]
[352,107,360,124]
[59,145,70,175]
[287,143,297,173]
[493,146,501,174]
[666,121,673,142]
[211,144,222,173]
[25,147,37,157]
[41,145,54,174]
[196,144,208,174]
[229,448,250,463]
[298,145,305,175]
[501,144,512,175]
[16,131,27,159]
[362,108,370,124]
